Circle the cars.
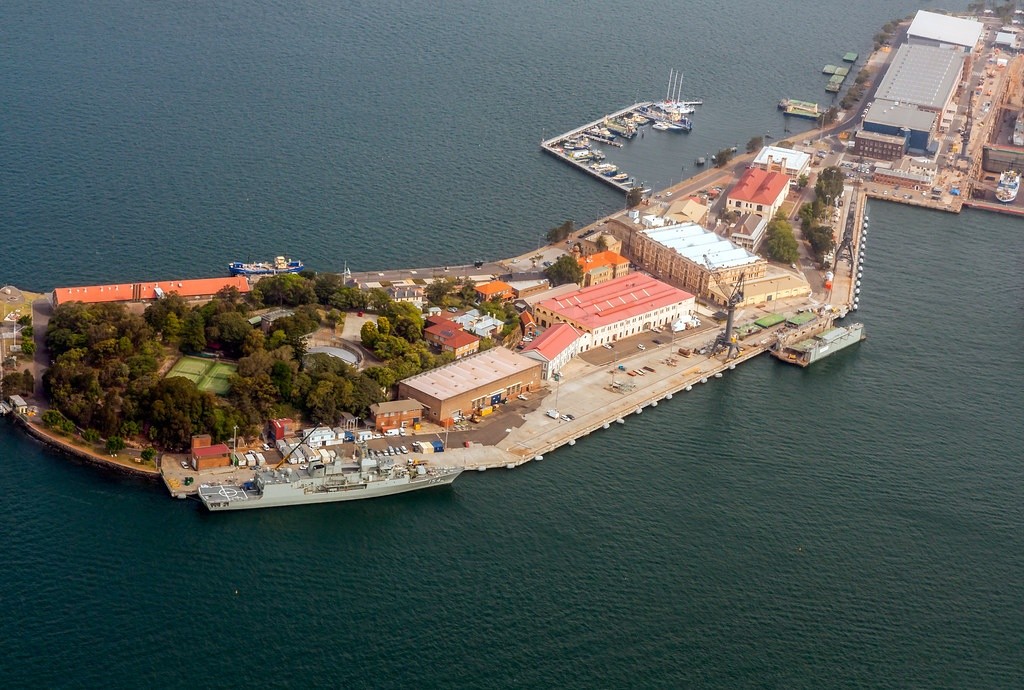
[383,450,389,456]
[375,450,382,457]
[666,192,672,197]
[181,461,189,469]
[567,240,572,244]
[567,414,575,419]
[655,195,662,199]
[400,446,409,453]
[395,447,401,455]
[578,220,609,238]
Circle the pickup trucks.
[518,395,528,400]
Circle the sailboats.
[655,67,695,114]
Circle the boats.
[995,170,1022,202]
[613,174,628,182]
[564,104,692,177]
[228,255,304,275]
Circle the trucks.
[399,428,406,436]
[408,459,429,466]
[546,409,559,419]
[384,429,399,436]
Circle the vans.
[561,415,567,420]
[262,443,269,451]
[611,381,624,387]
[638,343,645,350]
[388,446,395,456]
